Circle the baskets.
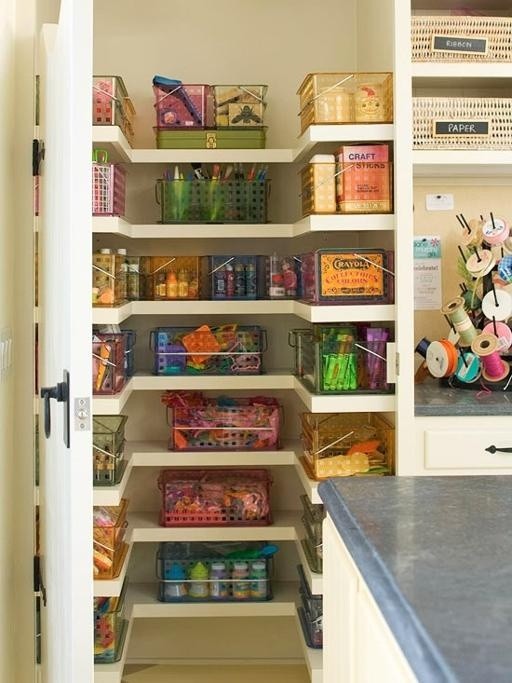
[36,576,129,664]
[92,254,127,308]
[149,325,267,375]
[33,160,126,215]
[300,494,323,573]
[90,247,393,307]
[300,410,394,480]
[288,328,387,395]
[296,161,392,213]
[296,564,322,649]
[296,71,393,133]
[153,179,271,223]
[157,468,275,527]
[413,96,512,150]
[92,330,137,395]
[93,74,136,148]
[165,397,284,451]
[36,499,129,580]
[154,541,274,601]
[151,83,268,130]
[92,414,128,485]
[411,15,511,63]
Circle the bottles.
[209,563,227,601]
[250,562,267,601]
[232,562,250,601]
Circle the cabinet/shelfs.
[27,116,409,680]
[396,1,512,469]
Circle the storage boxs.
[33,70,392,663]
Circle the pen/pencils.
[160,163,269,182]
[322,327,392,391]
